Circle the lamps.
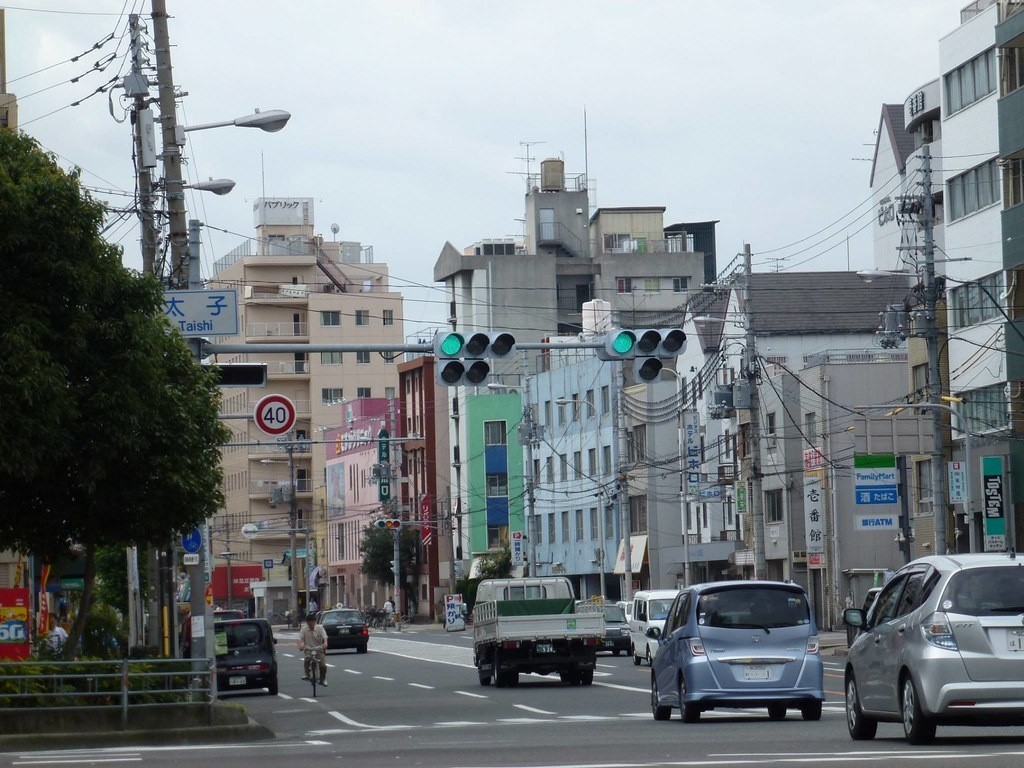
[922,543,930,547]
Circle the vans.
[630,589,705,666]
[616,601,632,623]
[645,579,826,724]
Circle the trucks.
[473,577,606,689]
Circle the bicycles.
[364,607,411,629]
[301,645,325,697]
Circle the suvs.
[841,550,1024,745]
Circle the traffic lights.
[429,329,519,389]
[605,328,687,383]
[374,519,403,529]
[198,363,268,388]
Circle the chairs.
[715,613,759,626]
[325,617,358,623]
[242,631,257,645]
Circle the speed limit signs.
[253,394,297,436]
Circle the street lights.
[132,109,293,659]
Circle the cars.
[139,602,282,696]
[315,608,369,653]
[577,604,634,656]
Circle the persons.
[215,604,223,612]
[384,597,396,615]
[300,614,329,686]
[310,598,317,616]
[45,613,68,655]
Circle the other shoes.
[321,680,328,687]
[302,675,310,681]
